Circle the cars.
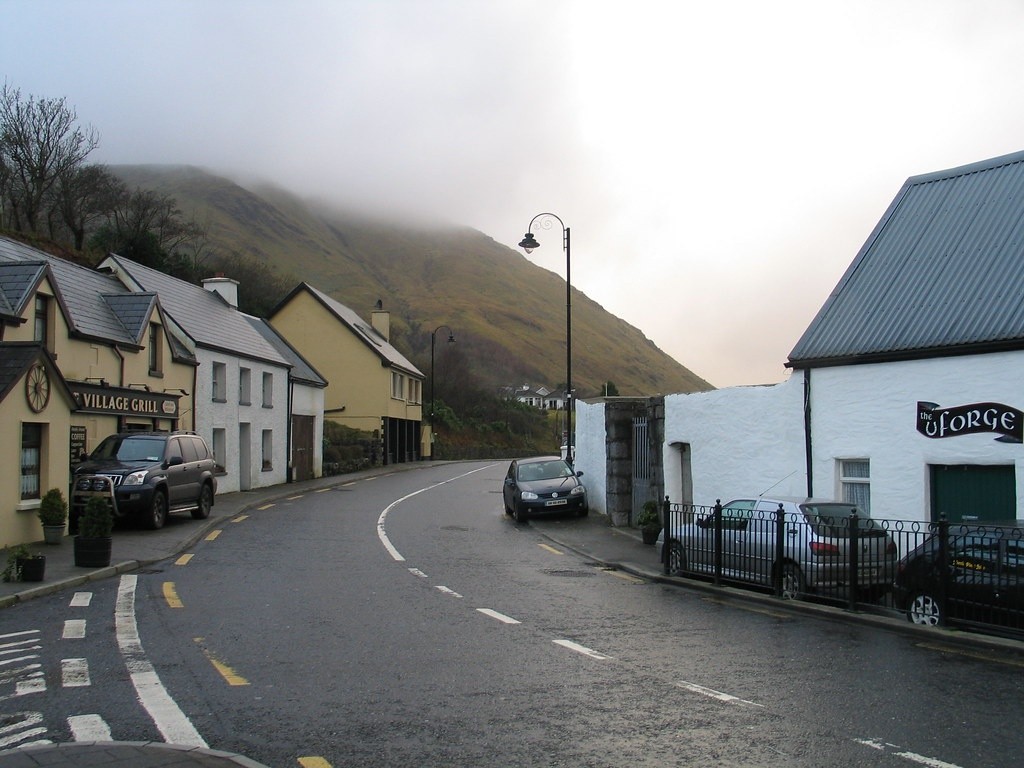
[888,520,1024,641]
[502,455,589,525]
[657,497,898,599]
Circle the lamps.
[85,377,109,388]
[128,383,153,392]
[163,389,190,396]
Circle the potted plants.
[36,488,69,544]
[0,545,47,581]
[73,493,113,568]
[636,500,662,545]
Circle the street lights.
[430,325,457,460]
[518,211,572,466]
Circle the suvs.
[68,429,218,532]
